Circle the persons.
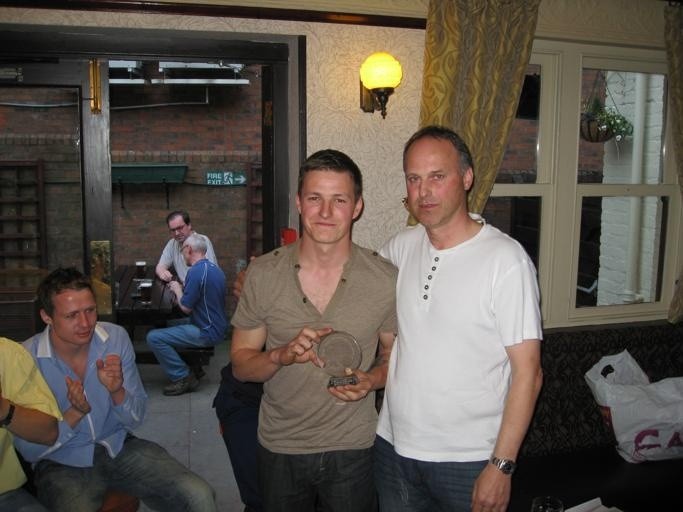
[231,124,544,512]
[146,234,227,396]
[155,210,217,287]
[8,266,221,512]
[0,336,64,512]
[230,149,399,511]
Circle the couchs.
[511,320,678,509]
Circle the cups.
[137,280,152,307]
[135,261,147,279]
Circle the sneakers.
[190,364,206,378]
[163,375,200,396]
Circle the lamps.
[356,50,405,121]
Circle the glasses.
[179,245,189,254]
[168,223,186,233]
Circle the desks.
[109,266,172,364]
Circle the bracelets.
[0,398,15,427]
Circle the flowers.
[582,100,633,142]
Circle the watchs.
[488,454,517,476]
